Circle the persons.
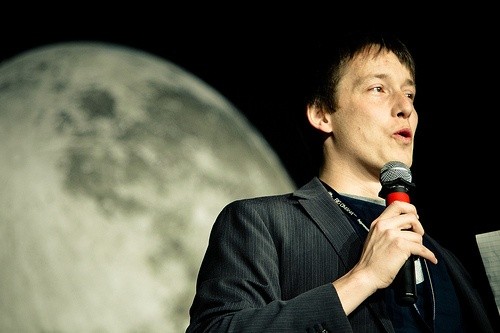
[185,33,500,333]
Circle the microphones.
[380,161,417,306]
